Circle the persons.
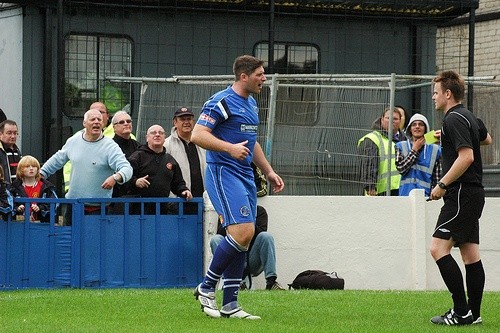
[0,165,18,223]
[107,110,143,215]
[356,106,408,196]
[61,102,137,198]
[10,154,60,223]
[393,104,440,146]
[39,109,133,227]
[397,112,442,198]
[161,106,209,216]
[208,205,287,291]
[189,55,285,322]
[125,124,193,215]
[428,70,494,325]
[0,120,24,184]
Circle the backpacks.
[287,270,344,290]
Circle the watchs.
[437,181,447,190]
[112,174,119,182]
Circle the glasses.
[113,119,133,125]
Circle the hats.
[173,107,195,119]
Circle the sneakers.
[267,282,286,291]
[194,282,221,318]
[474,316,483,325]
[238,282,249,291]
[220,306,261,320]
[430,308,474,326]
